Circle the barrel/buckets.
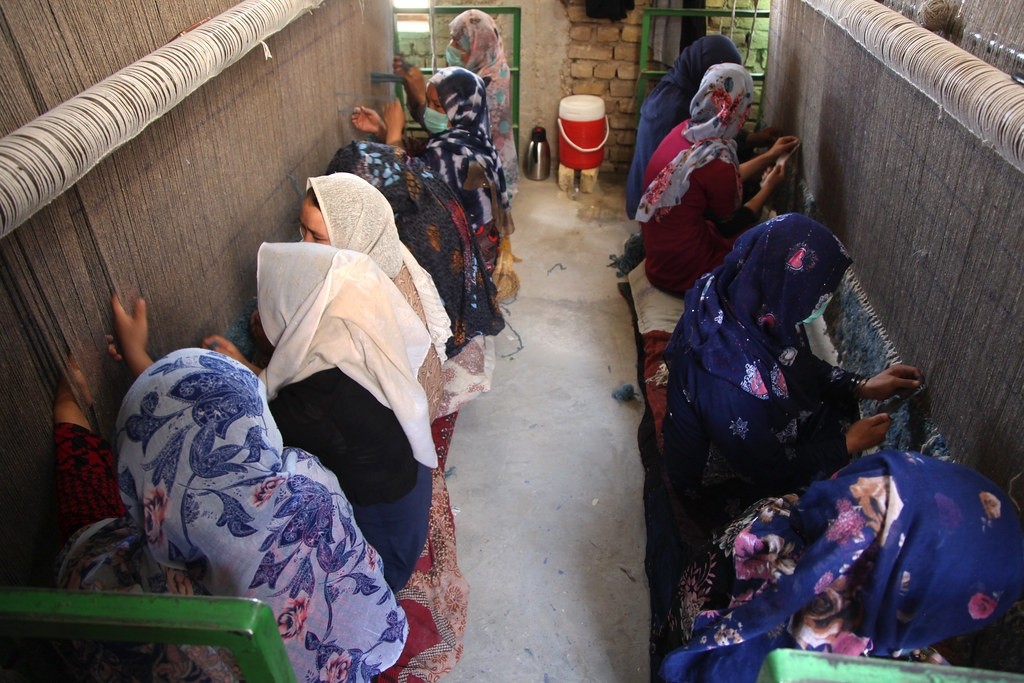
[558,94,609,170]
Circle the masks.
[802,295,834,324]
[445,44,467,68]
[423,106,450,135]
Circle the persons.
[251,171,454,428]
[626,35,781,219]
[656,451,1023,683]
[51,345,410,683]
[638,62,801,299]
[393,9,519,204]
[199,241,439,594]
[662,214,925,531]
[325,139,505,361]
[352,65,513,280]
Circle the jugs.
[525,126,551,181]
